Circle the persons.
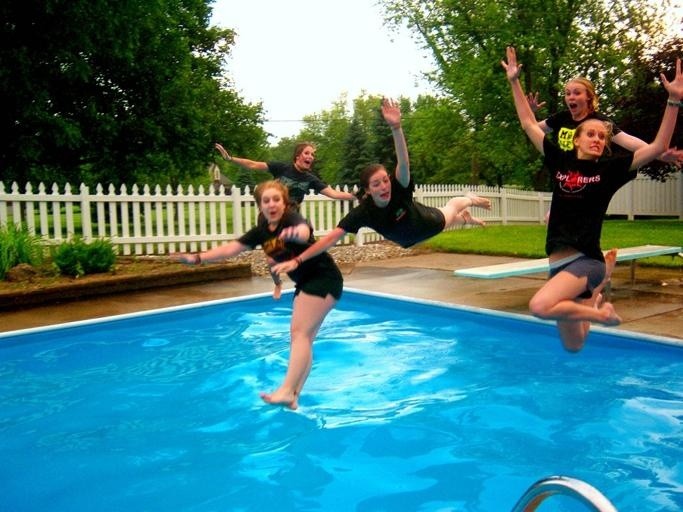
[499,44,682,353]
[166,178,344,410]
[523,78,682,312]
[212,142,357,299]
[271,97,491,276]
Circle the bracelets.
[668,99,681,106]
[292,257,303,266]
[229,156,232,162]
[193,254,200,265]
